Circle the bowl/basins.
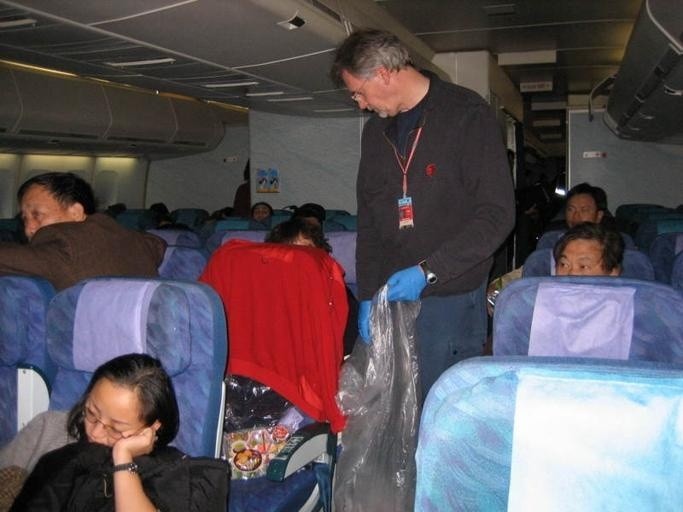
[230,439,247,453]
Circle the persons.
[265,222,360,359]
[553,221,624,277]
[149,202,326,231]
[0,353,178,511]
[1,173,167,292]
[511,146,564,254]
[332,28,519,410]
[562,182,605,226]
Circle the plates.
[232,448,262,472]
[275,432,289,440]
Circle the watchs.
[109,463,138,473]
[419,259,436,285]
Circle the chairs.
[1,207,362,512]
[411,353,683,512]
[523,201,681,286]
[484,278,683,362]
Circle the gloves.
[358,300,373,345]
[386,266,427,301]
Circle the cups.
[250,445,258,451]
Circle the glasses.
[78,404,148,440]
[352,80,367,102]
